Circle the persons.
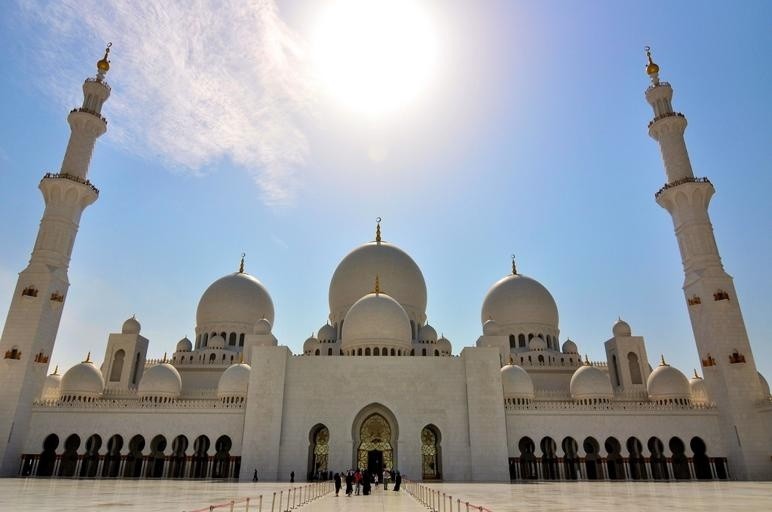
[289,471,295,482]
[308,466,402,497]
[252,469,258,481]
